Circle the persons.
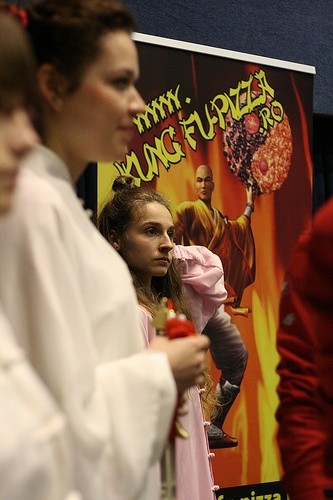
[274,198,333,500]
[171,161,253,448]
[0,10,84,500]
[21,0,215,500]
[98,178,229,500]
